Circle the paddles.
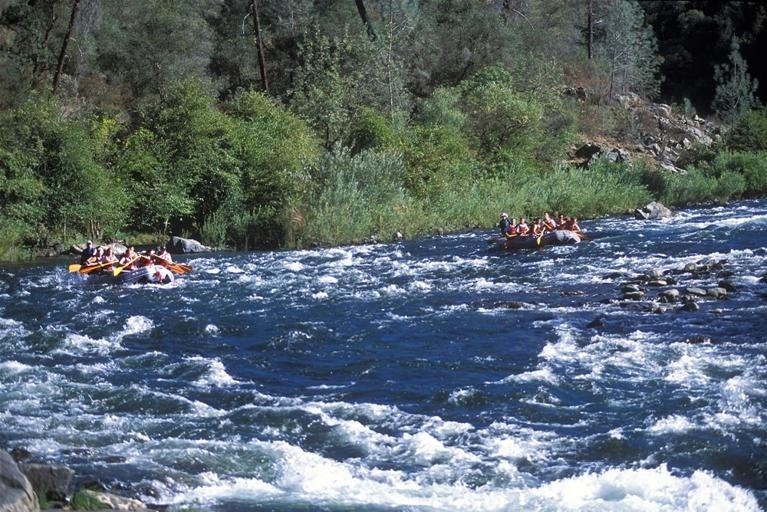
[537,227,546,245]
[114,256,141,276]
[148,253,192,274]
[576,233,592,240]
[68,264,100,272]
[80,261,120,272]
[504,233,522,248]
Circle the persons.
[497,212,580,238]
[80,240,170,271]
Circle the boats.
[86,264,174,285]
[495,230,582,250]
